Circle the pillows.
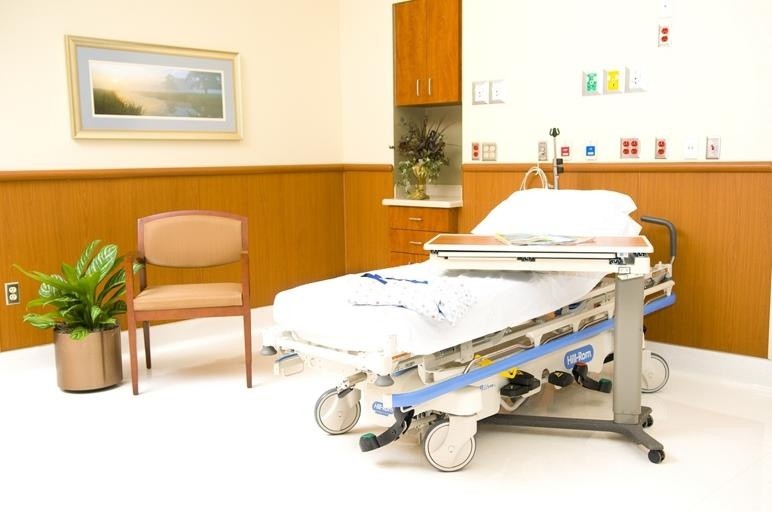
[470,188,643,239]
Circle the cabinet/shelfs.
[392,0,461,107]
[388,207,457,267]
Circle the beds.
[274,216,677,473]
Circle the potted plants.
[15,237,143,392]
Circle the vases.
[407,168,431,200]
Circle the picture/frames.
[66,34,243,145]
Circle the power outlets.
[472,79,508,103]
[581,66,648,95]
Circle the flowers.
[389,111,461,188]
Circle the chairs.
[125,210,254,396]
